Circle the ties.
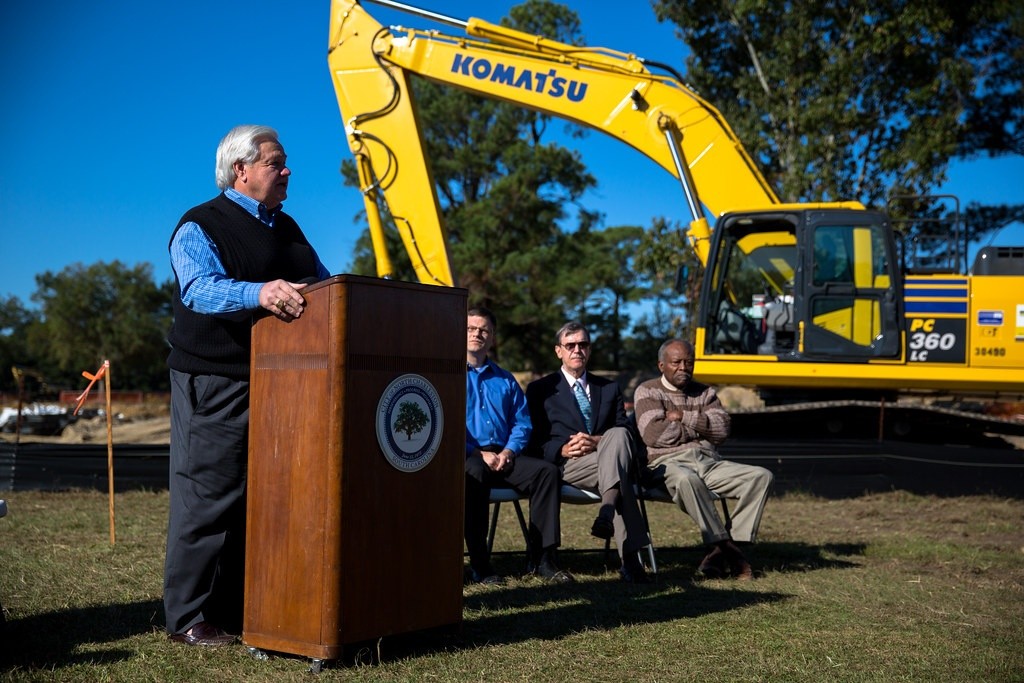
[573,381,593,434]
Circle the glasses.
[560,342,590,350]
[468,326,491,334]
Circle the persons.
[463,307,575,584]
[526,321,652,585]
[162,125,332,645]
[633,339,773,579]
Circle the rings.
[275,300,285,309]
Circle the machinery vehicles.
[327,0,1024,439]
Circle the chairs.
[561,485,646,571]
[625,409,737,573]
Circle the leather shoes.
[171,620,237,647]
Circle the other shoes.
[527,561,570,576]
[730,561,753,581]
[698,555,724,577]
[591,517,614,539]
[469,561,502,584]
[620,565,653,584]
